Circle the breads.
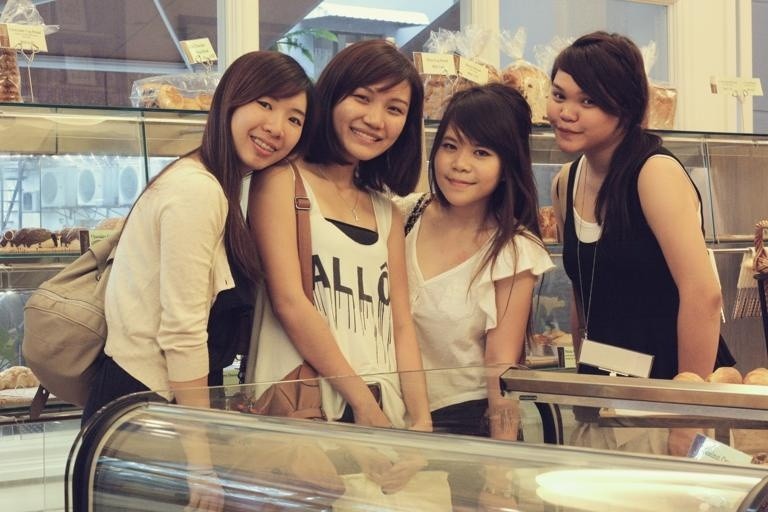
[641,87,677,129]
[1,217,124,253]
[1,364,37,391]
[0,49,22,102]
[504,62,551,123]
[530,328,576,355]
[140,82,213,110]
[540,206,560,239]
[459,62,500,91]
[424,63,458,119]
[675,364,768,386]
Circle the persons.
[546,30,738,458]
[80,52,318,511]
[389,85,555,442]
[244,42,433,432]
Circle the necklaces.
[319,161,361,220]
[576,156,612,335]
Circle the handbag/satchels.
[245,158,328,423]
[16,226,119,403]
